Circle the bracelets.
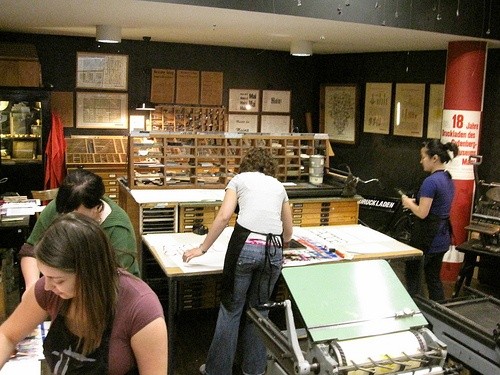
[199,244,206,254]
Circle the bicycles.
[344,164,416,244]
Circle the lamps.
[95,25,121,44]
[290,40,313,56]
[135,36,156,110]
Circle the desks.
[0,196,37,303]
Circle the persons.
[0,212,168,375]
[402,139,458,301]
[181,148,292,375]
[19,168,140,297]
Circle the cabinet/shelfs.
[116,130,362,286]
